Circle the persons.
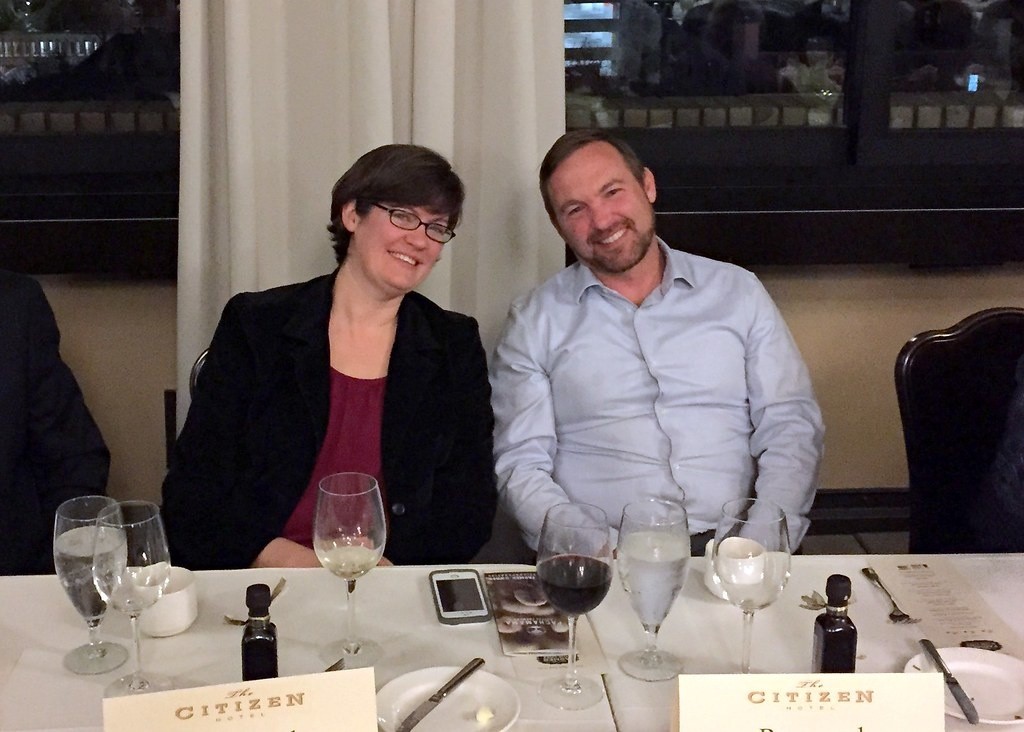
[489,128,826,557]
[0,268,111,575]
[161,143,498,571]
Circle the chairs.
[895,307,1024,553]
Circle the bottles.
[812,574,857,673]
[240,583,278,681]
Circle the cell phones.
[429,569,493,625]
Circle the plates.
[376,666,521,732]
[903,646,1024,725]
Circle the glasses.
[373,202,456,243]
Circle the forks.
[861,567,921,624]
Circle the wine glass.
[535,502,613,708]
[53,495,129,674]
[92,500,171,698]
[617,500,690,681]
[712,498,791,672]
[313,473,387,666]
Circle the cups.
[138,566,199,638]
[703,538,766,603]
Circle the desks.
[0,567,616,732]
[586,553,1024,732]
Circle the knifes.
[918,638,979,724]
[394,657,485,731]
